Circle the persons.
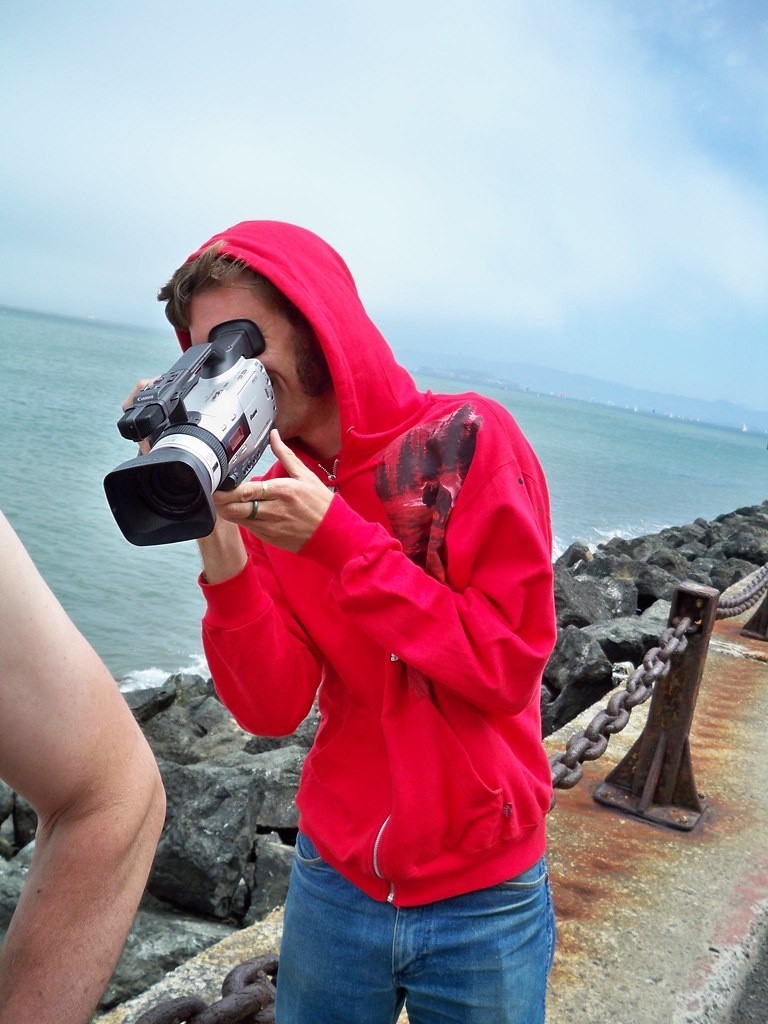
[122,221,558,1024]
[0,508,166,1024]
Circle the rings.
[246,500,258,520]
[260,481,267,501]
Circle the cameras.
[104,320,277,546]
